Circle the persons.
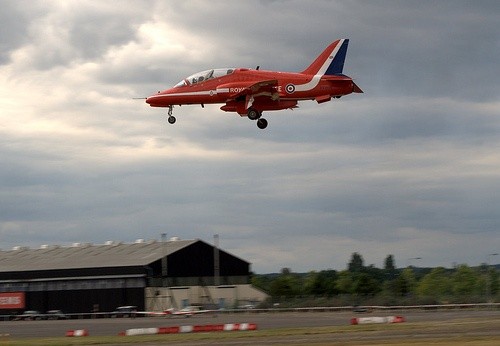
[198,76,204,82]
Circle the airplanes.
[145,39,363,130]
